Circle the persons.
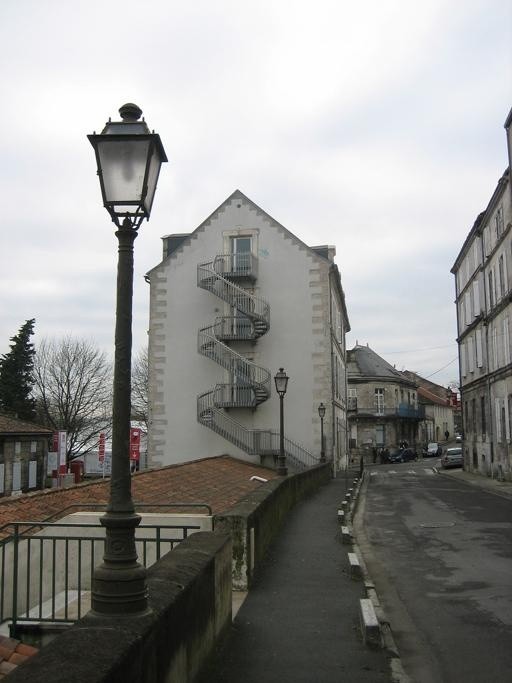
[444,429,449,441]
[371,445,390,464]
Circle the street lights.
[89,103,170,619]
[274,366,290,476]
[318,402,326,463]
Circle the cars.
[388,448,419,463]
[441,447,462,467]
[422,442,442,457]
[456,435,462,442]
